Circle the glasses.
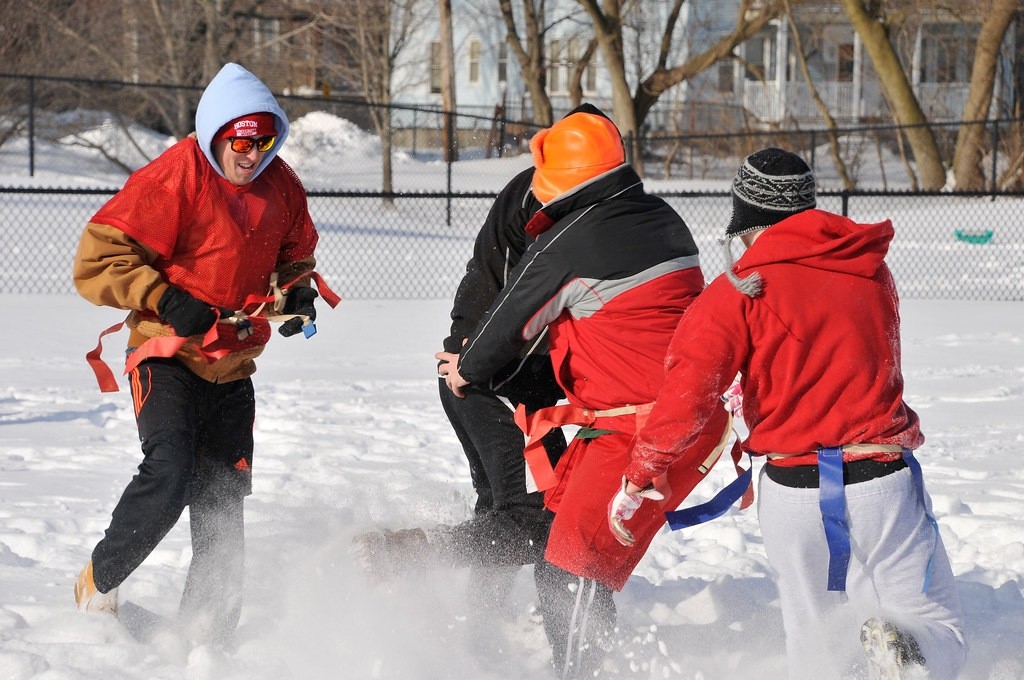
[226,135,277,153]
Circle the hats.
[562,103,610,119]
[717,147,816,298]
[211,112,278,145]
[530,111,626,206]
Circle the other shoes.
[859,616,926,680]
[73,560,118,615]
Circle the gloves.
[158,285,235,337]
[278,286,318,337]
[607,474,664,547]
[437,318,478,375]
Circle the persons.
[354,103,626,615]
[434,112,731,680]
[608,149,968,680]
[72,62,341,667]
